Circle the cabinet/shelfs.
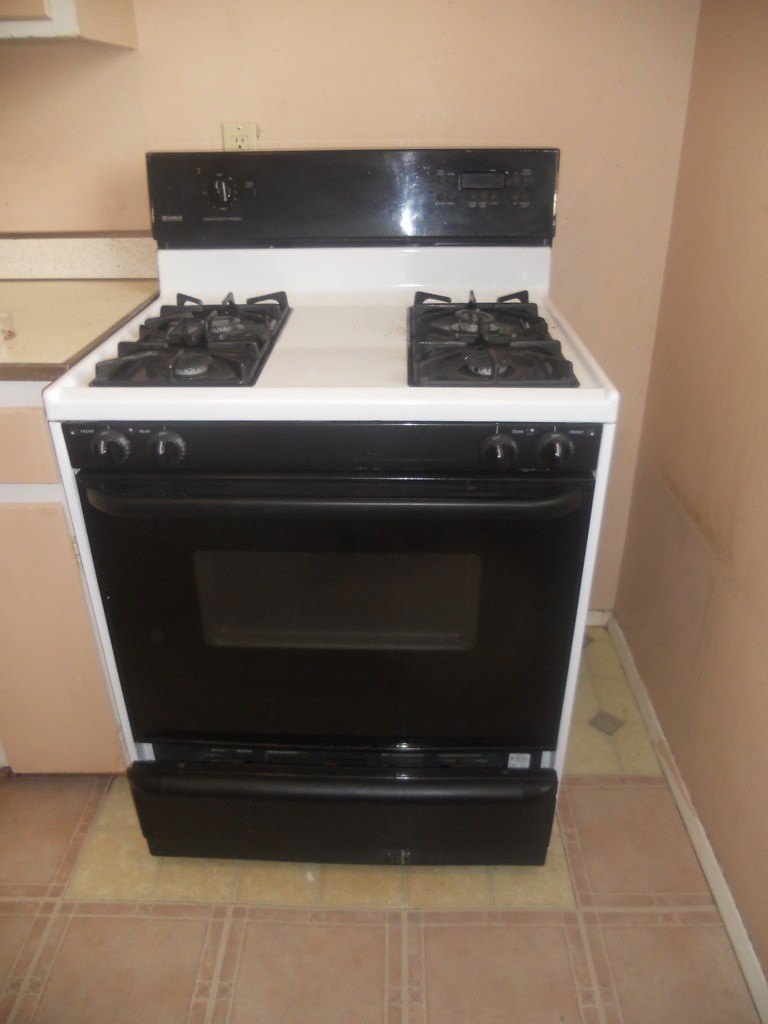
[1,0,139,52]
[0,374,134,776]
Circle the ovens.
[59,420,616,867]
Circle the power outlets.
[222,122,257,152]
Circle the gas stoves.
[40,148,618,421]
